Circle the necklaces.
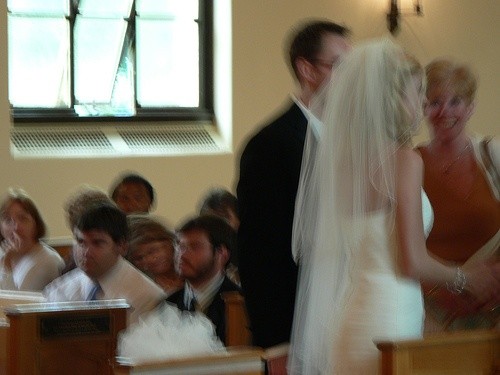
[441,144,469,175]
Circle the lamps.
[387,0,421,33]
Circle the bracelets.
[455,270,466,296]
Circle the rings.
[491,307,496,312]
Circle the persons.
[0,193,65,292]
[63,174,237,296]
[42,206,167,325]
[285,38,478,375]
[152,215,243,352]
[418,58,500,332]
[234,18,356,350]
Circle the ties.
[189,297,196,313]
[86,284,103,302]
